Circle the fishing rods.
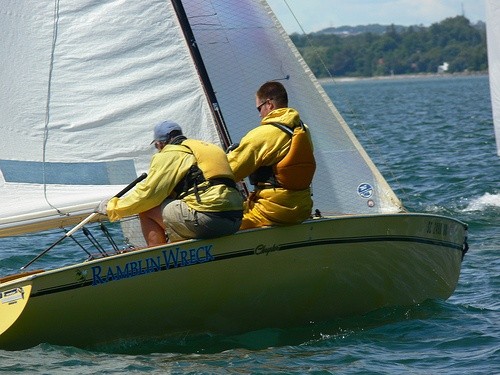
[20,173,148,269]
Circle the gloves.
[93,199,107,216]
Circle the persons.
[92,120,244,247]
[227,81,316,231]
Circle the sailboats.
[0,0,469,356]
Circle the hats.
[150,120,181,146]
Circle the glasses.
[257,98,272,112]
